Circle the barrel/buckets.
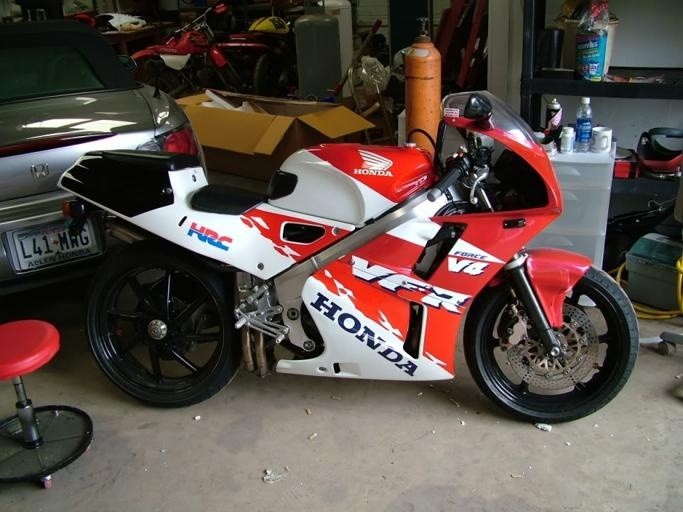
[573,18,620,82]
[535,28,565,69]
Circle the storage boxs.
[523,143,615,269]
[166,87,377,186]
[624,233,683,312]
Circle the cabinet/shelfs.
[520,1,682,268]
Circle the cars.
[0,18,208,299]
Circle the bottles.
[575,96,593,153]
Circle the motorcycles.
[129,4,297,92]
[57,91,641,424]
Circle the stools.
[0,319,94,489]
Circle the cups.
[592,126,613,152]
